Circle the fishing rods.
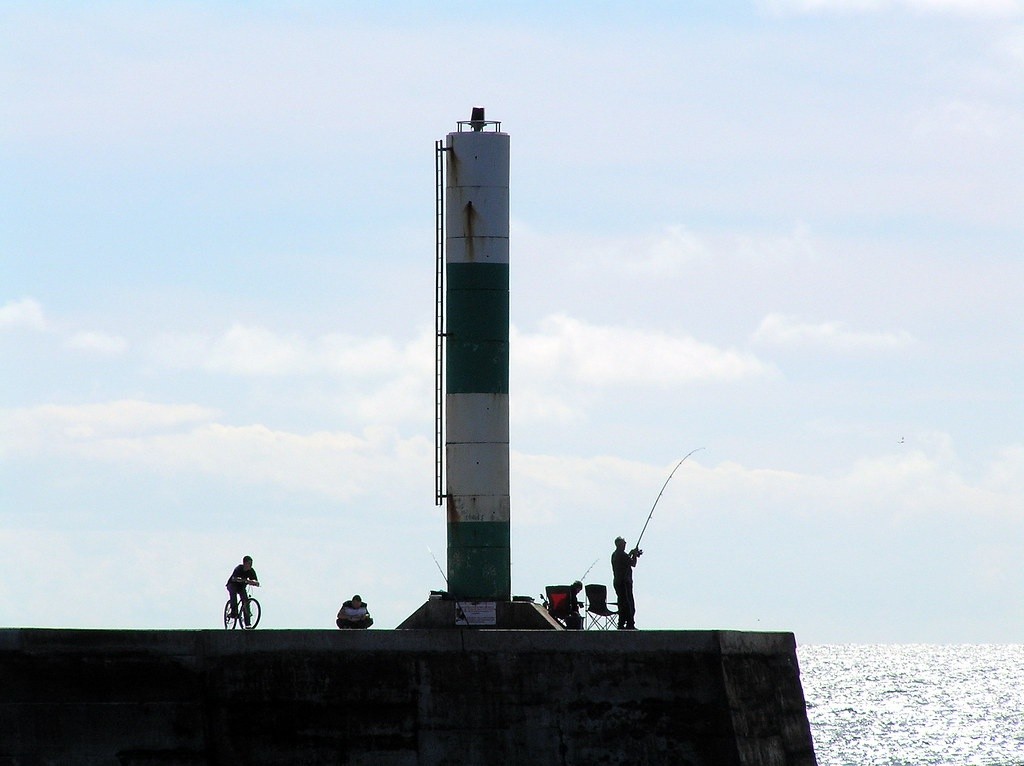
[632,445,706,559]
[580,558,599,581]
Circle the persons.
[336,595,373,629]
[555,581,584,628]
[611,537,641,629]
[225,556,260,629]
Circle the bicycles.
[223,579,261,630]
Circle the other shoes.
[230,613,242,618]
[618,626,625,630]
[246,624,252,629]
[626,625,636,629]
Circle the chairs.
[546,585,583,631]
[585,584,619,630]
